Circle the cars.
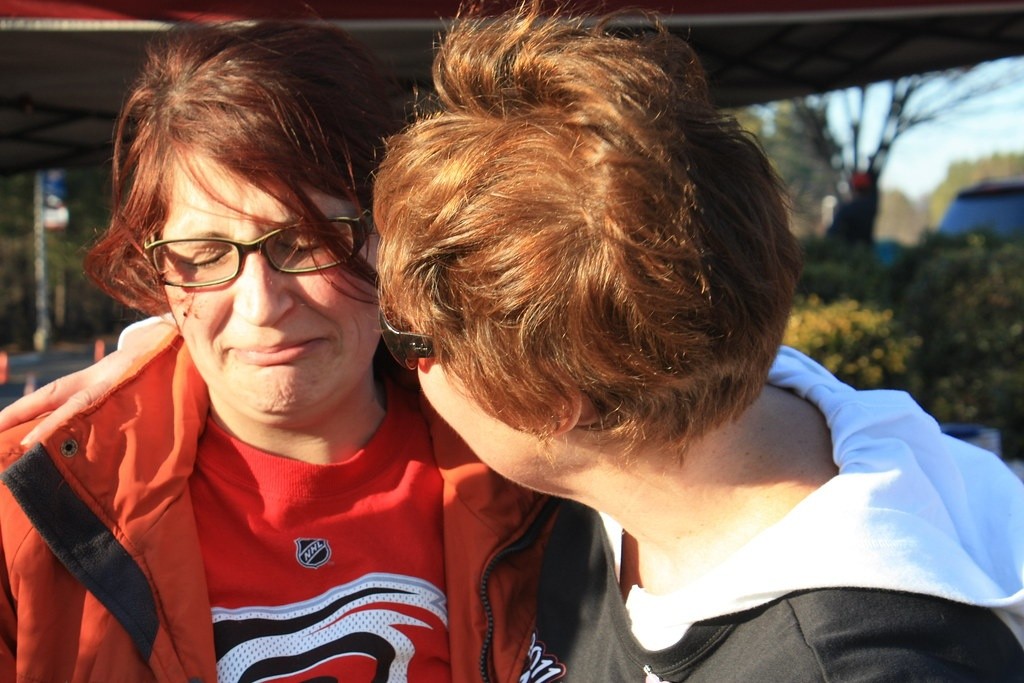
[936,174,1024,238]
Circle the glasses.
[142,205,374,288]
[378,308,437,370]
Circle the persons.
[0,1,564,683]
[824,169,880,245]
[372,1,1023,683]
